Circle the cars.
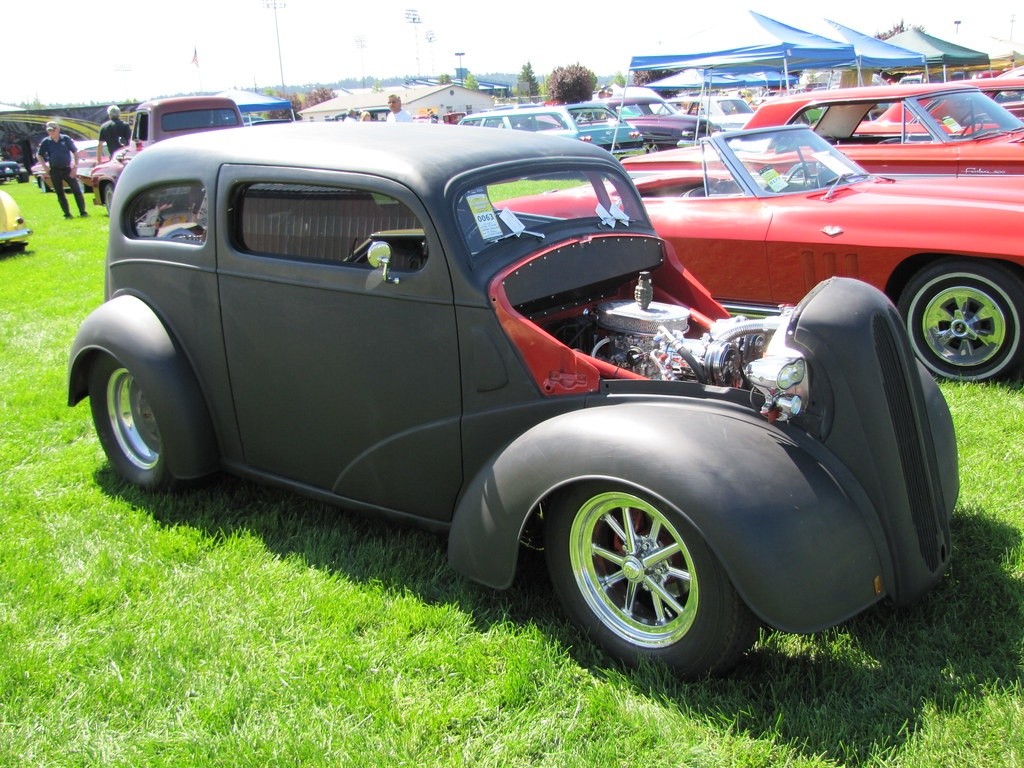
[454,66,1024,384]
[32,96,243,217]
[65,121,960,682]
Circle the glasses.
[388,101,397,104]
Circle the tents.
[216,88,295,126]
[608,10,1024,154]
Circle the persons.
[386,94,411,121]
[598,90,605,99]
[96,105,131,163]
[360,111,371,121]
[428,110,438,123]
[344,108,357,123]
[36,122,88,218]
[17,134,34,167]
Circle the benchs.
[241,183,425,264]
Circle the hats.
[47,121,58,130]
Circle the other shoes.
[80,211,89,217]
[63,216,73,220]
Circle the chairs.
[680,186,718,198]
[715,180,743,194]
